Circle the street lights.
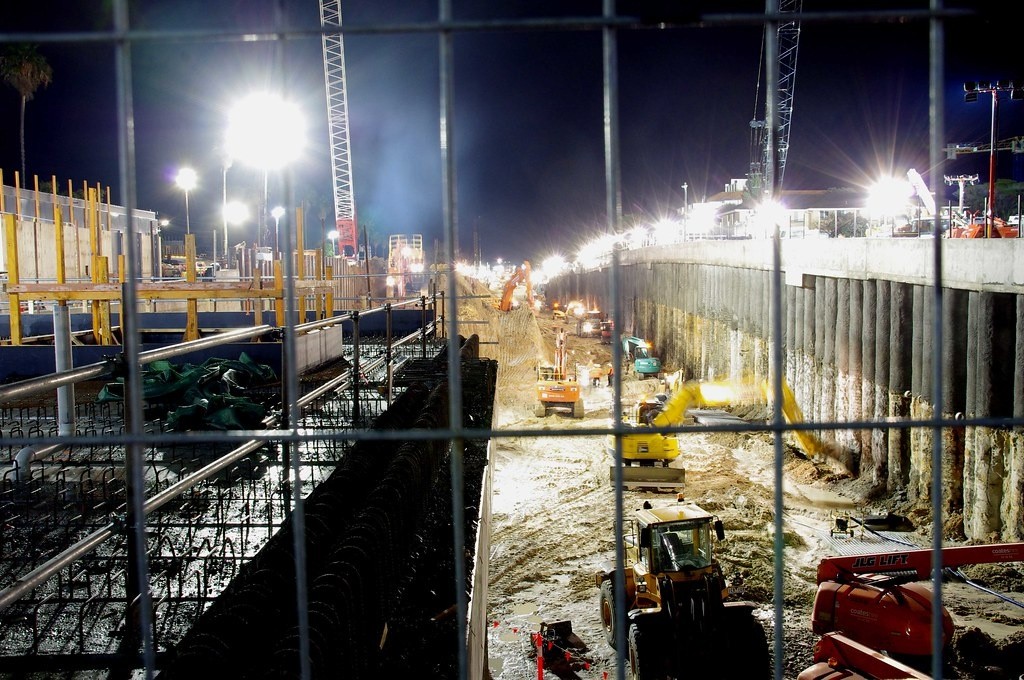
[964,78,1024,238]
[273,209,282,252]
[680,182,688,242]
[944,174,980,215]
[329,232,335,251]
[178,170,195,234]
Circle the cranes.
[943,136,1024,160]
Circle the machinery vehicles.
[622,336,664,380]
[484,261,540,316]
[594,503,771,680]
[533,328,584,418]
[600,322,614,345]
[609,375,824,494]
[576,311,604,338]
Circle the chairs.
[661,532,687,569]
